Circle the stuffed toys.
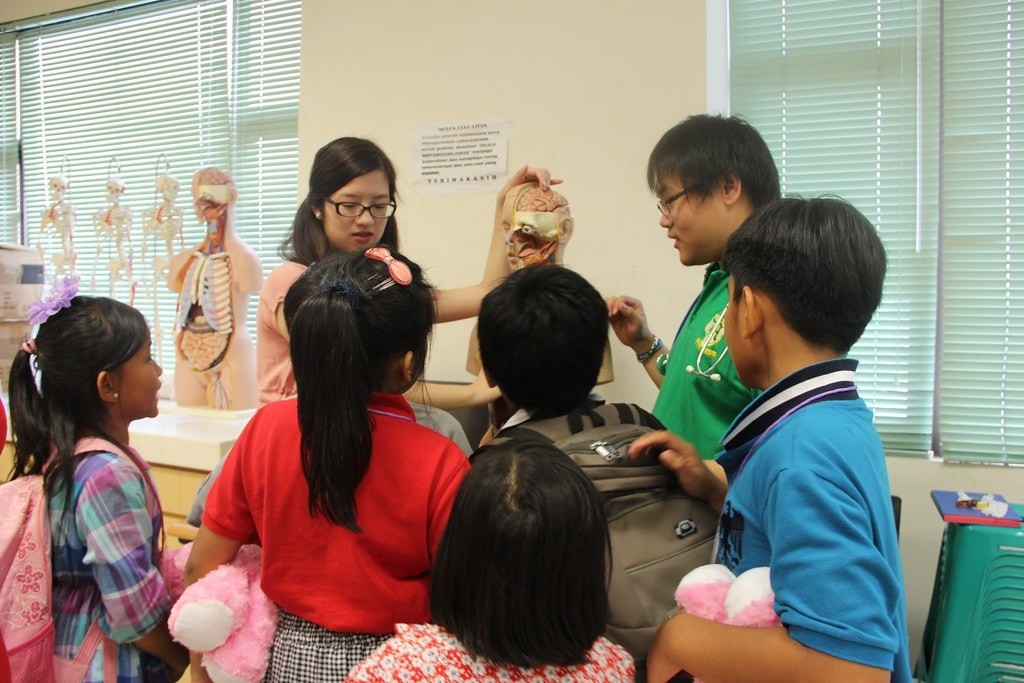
[161,540,278,683]
[675,564,781,681]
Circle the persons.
[6,294,186,683]
[630,197,914,683]
[258,137,501,455]
[465,183,613,383]
[164,169,262,408]
[185,246,469,683]
[604,113,784,486]
[466,266,669,452]
[339,441,635,683]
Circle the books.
[930,490,1022,527]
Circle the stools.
[912,504,1023,682]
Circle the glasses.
[657,182,698,216]
[323,196,397,218]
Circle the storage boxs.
[1,243,43,394]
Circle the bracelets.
[638,336,663,363]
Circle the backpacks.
[1,438,154,683]
[517,402,719,683]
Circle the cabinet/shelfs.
[0,404,256,683]
